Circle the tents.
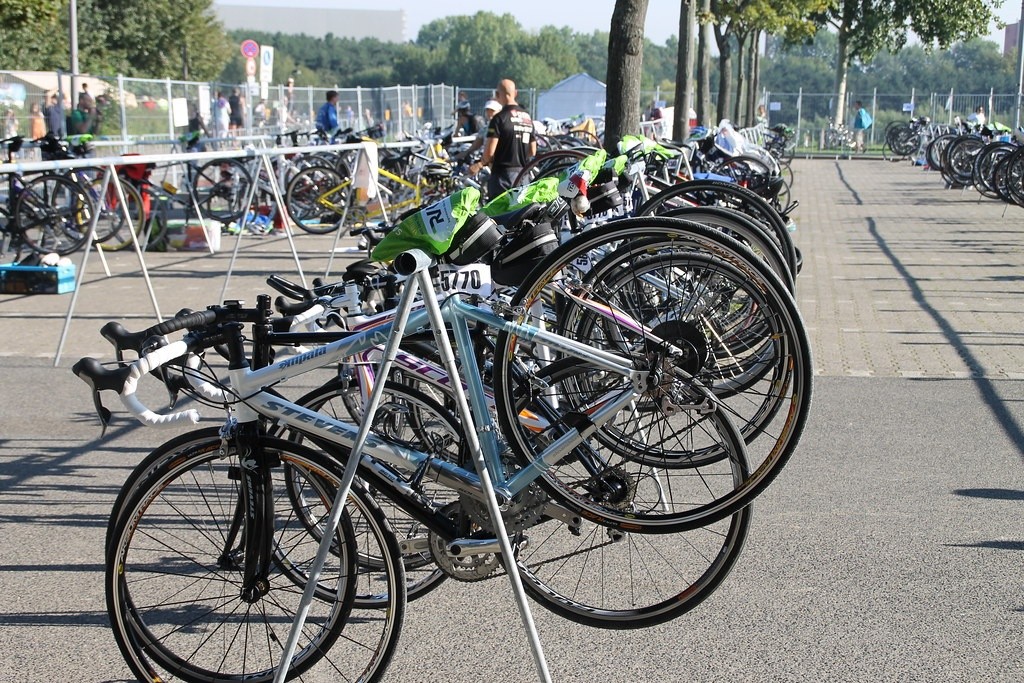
[535,71,609,128]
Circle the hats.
[483,100,502,113]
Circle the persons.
[853,101,867,156]
[755,104,769,130]
[967,106,985,126]
[467,78,537,204]
[444,100,504,172]
[1,66,433,149]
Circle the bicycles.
[0,96,1024,682]
